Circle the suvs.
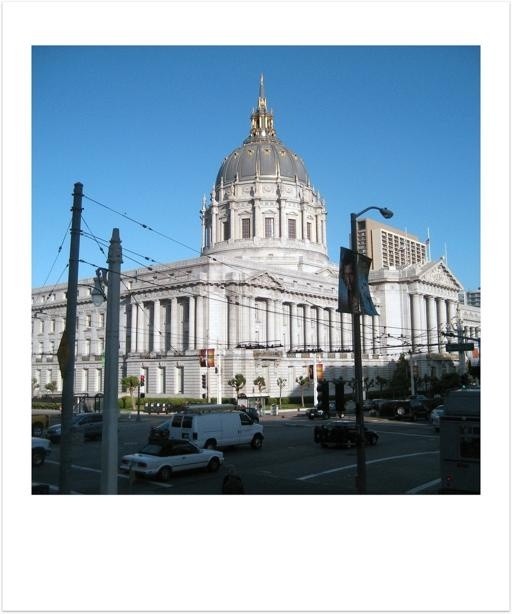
[308,400,339,420]
[314,419,379,449]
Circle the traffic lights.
[140,375,144,386]
[202,374,206,389]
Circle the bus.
[437,387,480,495]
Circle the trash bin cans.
[270,404,278,416]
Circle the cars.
[32,411,52,468]
[46,411,103,443]
[362,394,440,422]
[119,437,224,481]
[148,417,171,447]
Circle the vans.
[167,410,265,450]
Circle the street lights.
[351,204,394,476]
[89,227,124,495]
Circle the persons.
[256,398,262,416]
[342,252,364,314]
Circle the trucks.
[183,404,260,424]
